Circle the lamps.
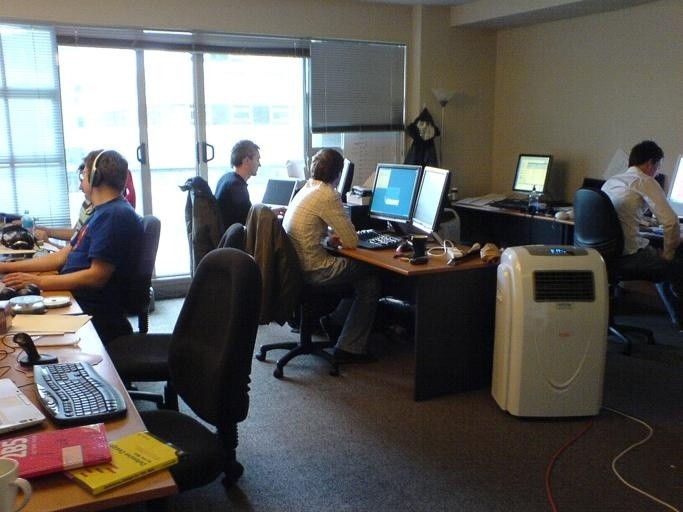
[428,85,458,165]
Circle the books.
[0,423,112,479]
[67,429,192,495]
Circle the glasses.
[78,168,91,181]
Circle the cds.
[43,296,71,308]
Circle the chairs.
[249,203,343,380]
[182,180,225,252]
[123,246,264,509]
[569,188,662,351]
[82,210,166,411]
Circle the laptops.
[260,179,297,219]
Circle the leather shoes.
[332,347,377,365]
[316,313,340,345]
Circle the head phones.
[520,199,552,215]
[0,226,34,249]
[89,150,113,186]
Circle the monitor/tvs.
[666,154,683,216]
[336,157,354,203]
[412,165,451,242]
[512,154,553,202]
[368,162,423,238]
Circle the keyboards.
[356,229,403,250]
[33,361,127,427]
[491,198,545,211]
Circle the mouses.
[396,244,413,252]
[545,207,556,216]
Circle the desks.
[0,239,177,510]
[450,192,575,249]
[636,225,683,254]
[317,227,498,403]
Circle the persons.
[281,146,377,369]
[213,140,286,232]
[2,149,143,342]
[12,164,92,251]
[600,141,683,302]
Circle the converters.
[447,248,463,258]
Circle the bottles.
[446,187,458,202]
[527,187,539,213]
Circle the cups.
[20,210,34,239]
[0,457,30,511]
[409,235,428,256]
[36,241,42,246]
